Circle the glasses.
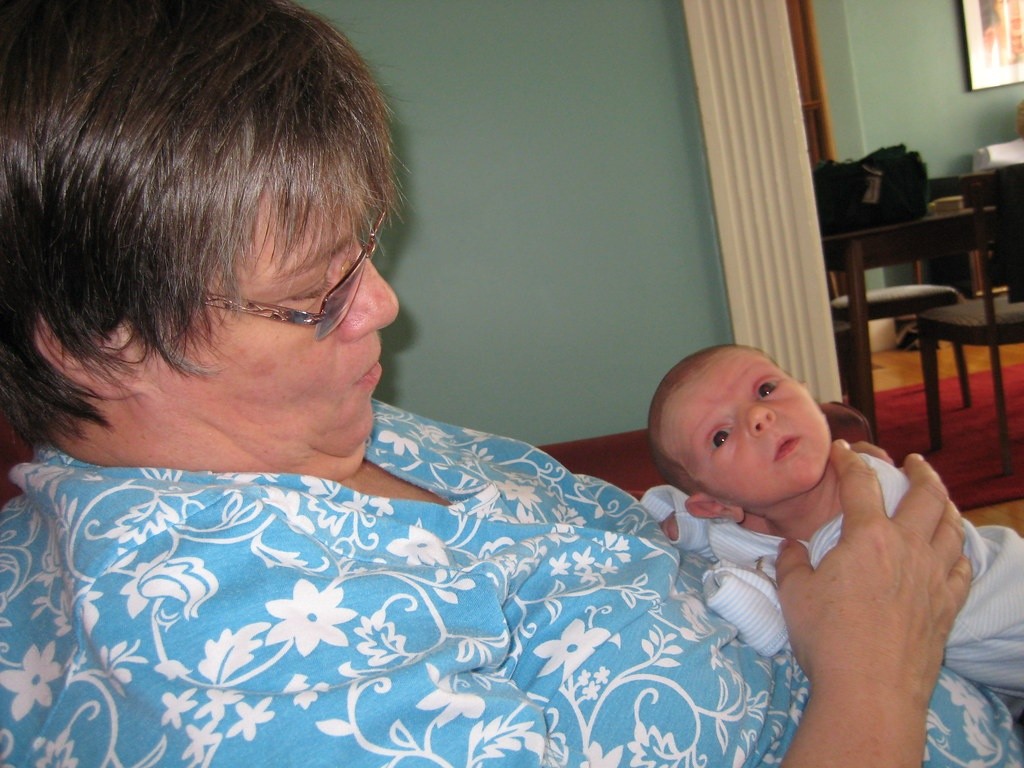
[190,205,389,337]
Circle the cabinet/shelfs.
[927,171,1007,297]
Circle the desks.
[821,209,994,448]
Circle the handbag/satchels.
[816,142,925,233]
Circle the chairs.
[916,163,1024,474]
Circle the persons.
[0,1,1022,766]
[646,344,1024,719]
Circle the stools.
[832,287,969,417]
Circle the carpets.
[843,363,1023,511]
